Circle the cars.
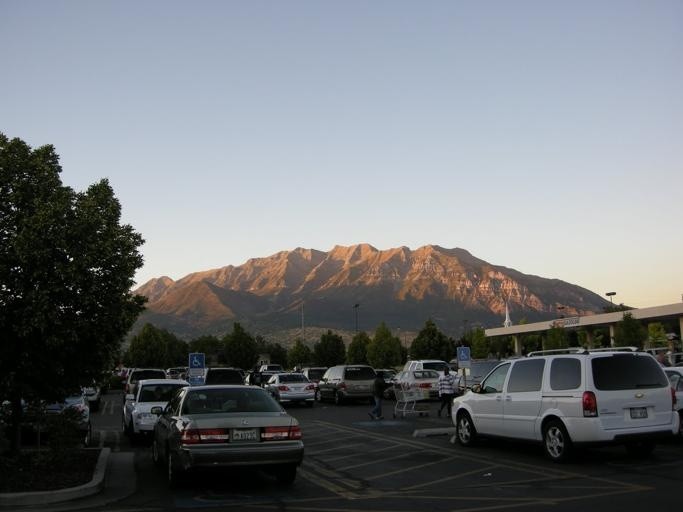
[451,346,683,463]
[0,377,100,448]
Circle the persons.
[369,373,392,420]
[666,350,676,367]
[437,366,454,417]
[152,387,162,400]
[251,369,262,387]
